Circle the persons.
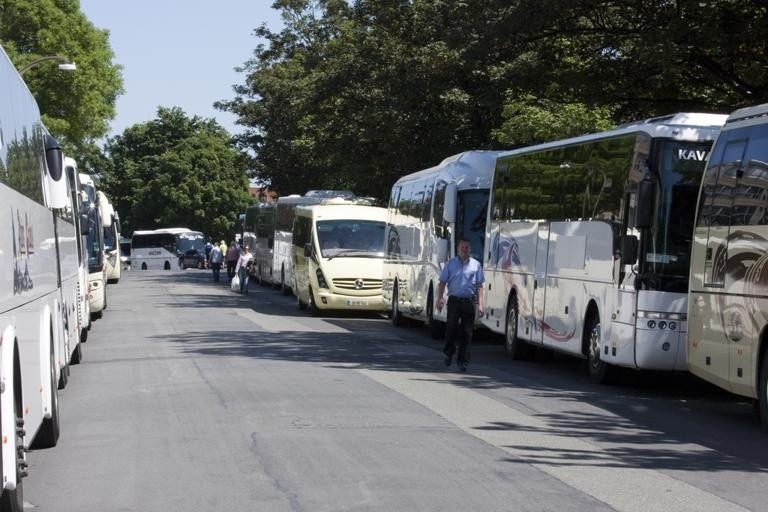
[203,236,244,283]
[435,239,486,373]
[234,244,254,296]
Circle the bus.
[478,110,731,384]
[235,190,379,296]
[130,228,206,270]
[382,149,504,340]
[685,102,768,434]
[0,41,131,512]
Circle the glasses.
[245,247,249,250]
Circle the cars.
[178,248,209,270]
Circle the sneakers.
[460,364,470,372]
[444,353,452,367]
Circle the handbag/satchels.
[230,276,240,291]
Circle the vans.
[289,203,388,316]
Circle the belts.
[449,295,476,302]
